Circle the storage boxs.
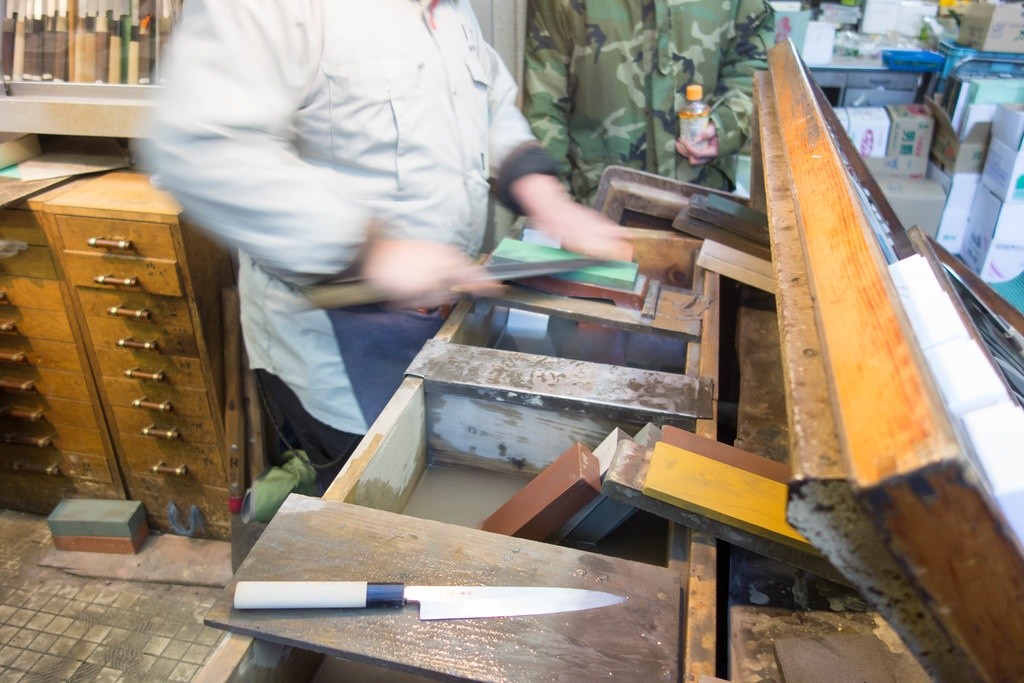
[770,0,1023,313]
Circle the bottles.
[679,85,710,150]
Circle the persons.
[125,0,638,489]
[519,0,777,208]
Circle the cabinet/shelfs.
[0,169,232,542]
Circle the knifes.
[310,259,613,307]
[234,581,631,621]
